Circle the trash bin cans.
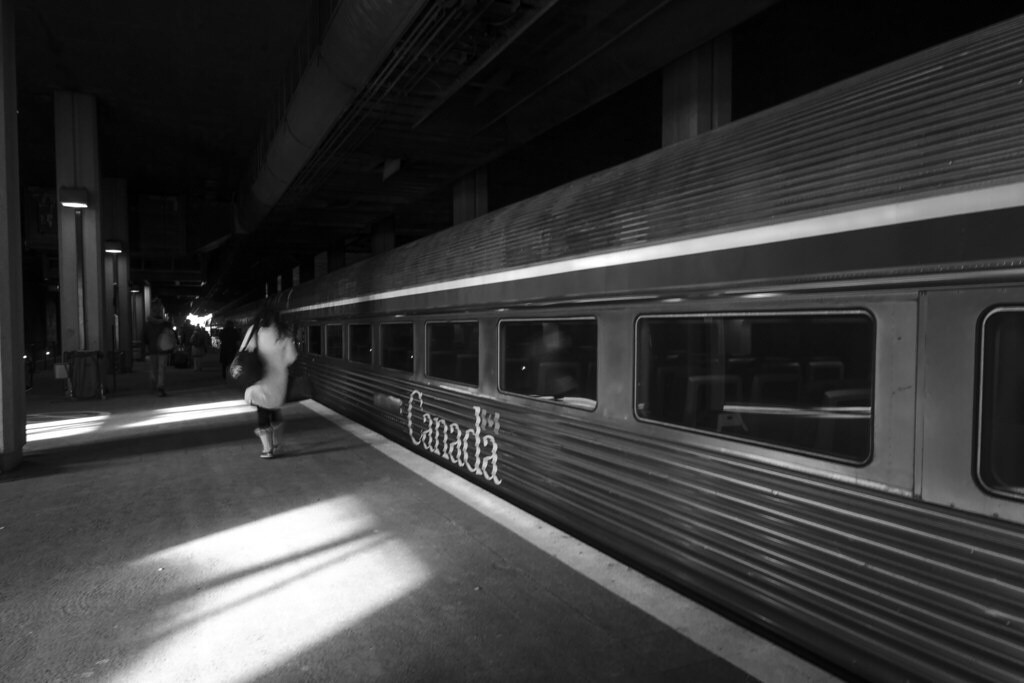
[63,350,105,400]
[132,340,143,360]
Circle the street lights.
[130,285,140,341]
[59,185,91,351]
[104,241,123,352]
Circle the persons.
[228,306,298,460]
[189,325,209,373]
[140,304,243,398]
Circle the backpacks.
[183,326,194,338]
[192,334,204,347]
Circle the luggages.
[175,345,190,368]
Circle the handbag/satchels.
[158,328,177,352]
[225,351,263,399]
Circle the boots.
[254,428,274,458]
[270,422,284,451]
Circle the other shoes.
[148,381,156,393]
[157,388,167,398]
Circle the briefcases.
[139,353,159,382]
[285,375,312,402]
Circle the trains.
[209,12,1024,682]
